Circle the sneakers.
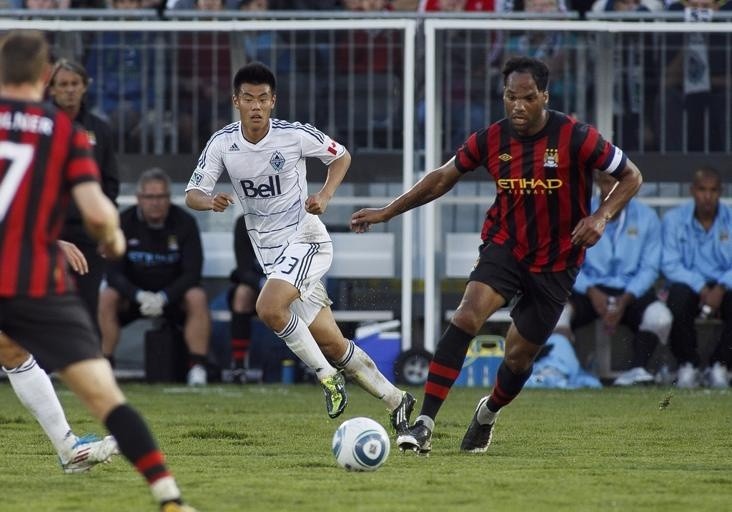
[158,501,199,512]
[389,391,417,432]
[186,365,251,385]
[62,434,123,475]
[396,421,432,458]
[322,371,348,418]
[459,397,502,455]
[614,362,730,390]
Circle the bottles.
[604,297,617,335]
[282,360,296,384]
[700,304,710,318]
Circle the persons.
[92,167,210,386]
[351,56,645,455]
[1,0,731,172]
[0,32,195,511]
[661,166,731,388]
[47,60,120,352]
[0,240,121,475]
[184,61,416,433]
[571,167,674,388]
[227,214,318,384]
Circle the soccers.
[332,418,390,472]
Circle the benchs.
[91,303,732,383]
